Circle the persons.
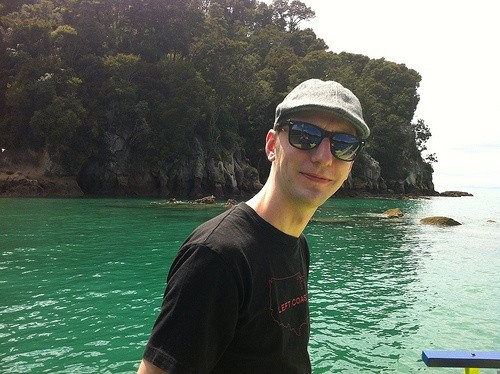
[137,79,370,374]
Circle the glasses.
[277,120,366,162]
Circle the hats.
[274,78,371,143]
